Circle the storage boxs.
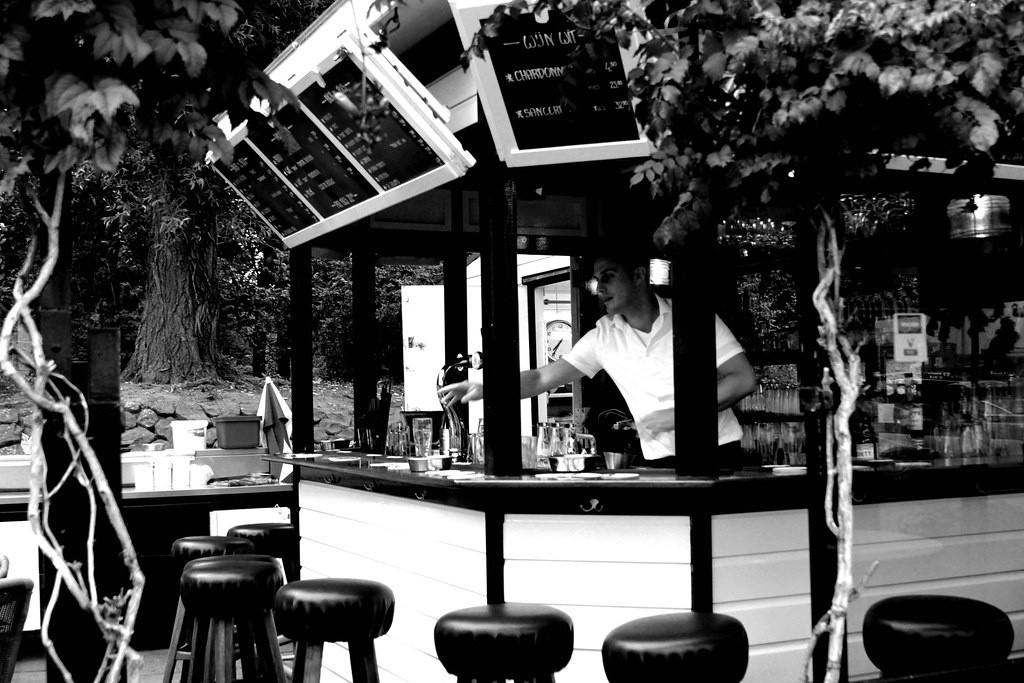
[213,416,262,449]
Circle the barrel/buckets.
[170,419,210,451]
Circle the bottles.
[477,418,484,431]
[842,286,920,330]
[735,365,1024,462]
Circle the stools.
[863,594,1015,683]
[275,578,396,683]
[162,522,297,683]
[433,602,574,683]
[600,611,750,683]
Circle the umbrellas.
[257,376,292,455]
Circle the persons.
[437,241,756,469]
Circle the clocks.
[546,320,572,363]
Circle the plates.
[535,472,640,482]
[275,452,323,460]
[894,463,932,467]
[329,456,362,462]
[762,464,808,474]
[868,458,893,462]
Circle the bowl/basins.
[140,443,166,450]
[547,455,595,471]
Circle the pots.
[313,439,353,450]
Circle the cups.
[521,422,596,469]
[413,418,433,456]
[408,457,429,472]
[467,431,487,467]
[132,453,212,490]
[432,456,452,469]
[603,450,631,469]
[353,424,411,456]
